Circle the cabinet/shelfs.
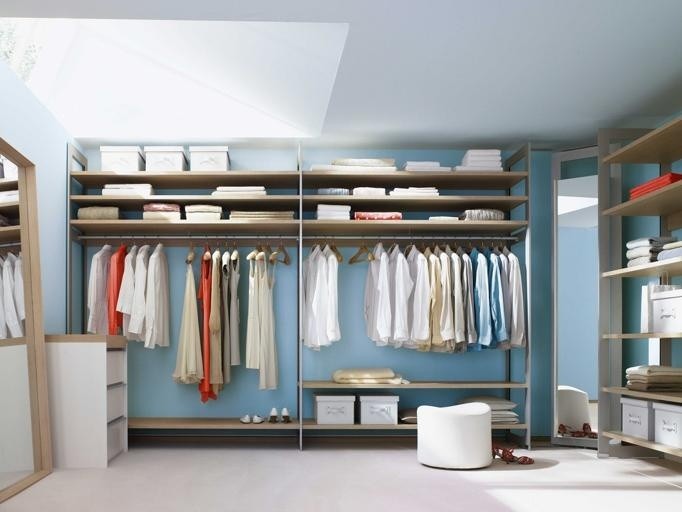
[44,332,128,469]
[298,138,536,453]
[68,138,299,452]
[600,110,682,463]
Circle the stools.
[557,384,592,432]
[414,399,494,470]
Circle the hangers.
[1,242,23,262]
[99,233,293,266]
[312,235,512,263]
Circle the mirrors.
[551,144,602,450]
[0,134,54,504]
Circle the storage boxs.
[358,392,401,425]
[99,144,235,172]
[1,155,18,179]
[314,394,357,425]
[650,287,682,334]
[650,403,682,453]
[618,396,654,440]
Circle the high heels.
[559,424,566,436]
[503,447,534,464]
[281,407,290,423]
[492,447,504,459]
[572,423,598,438]
[268,407,278,423]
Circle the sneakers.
[240,414,251,424]
[253,414,264,424]
